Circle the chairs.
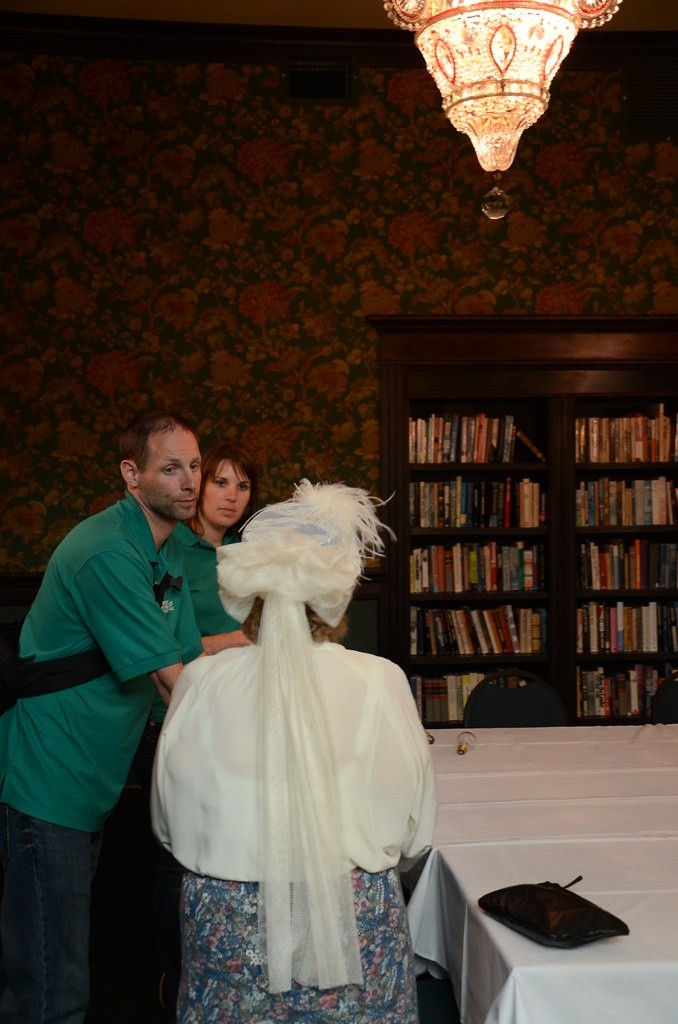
[464,668,567,728]
[652,671,678,725]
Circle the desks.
[405,724,677,1024]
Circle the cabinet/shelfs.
[367,314,678,729]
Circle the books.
[404,399,678,727]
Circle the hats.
[211,476,399,995]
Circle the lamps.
[382,0,624,219]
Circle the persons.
[135,443,257,846]
[147,498,441,1022]
[0,407,211,1023]
